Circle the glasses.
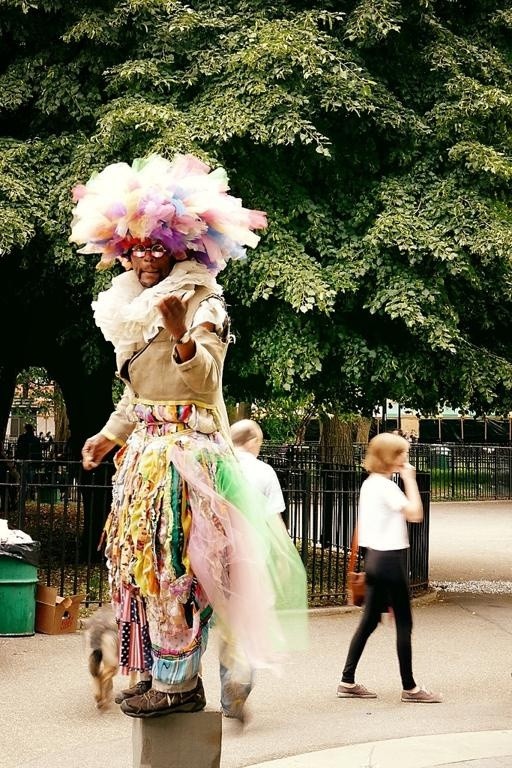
[131,244,168,258]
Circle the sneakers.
[115,678,207,717]
[336,681,378,698]
[401,686,442,703]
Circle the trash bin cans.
[0,542,41,636]
[431,447,450,470]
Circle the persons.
[335,434,447,703]
[81,216,254,719]
[16,423,42,501]
[230,419,286,656]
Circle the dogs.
[83,602,149,715]
